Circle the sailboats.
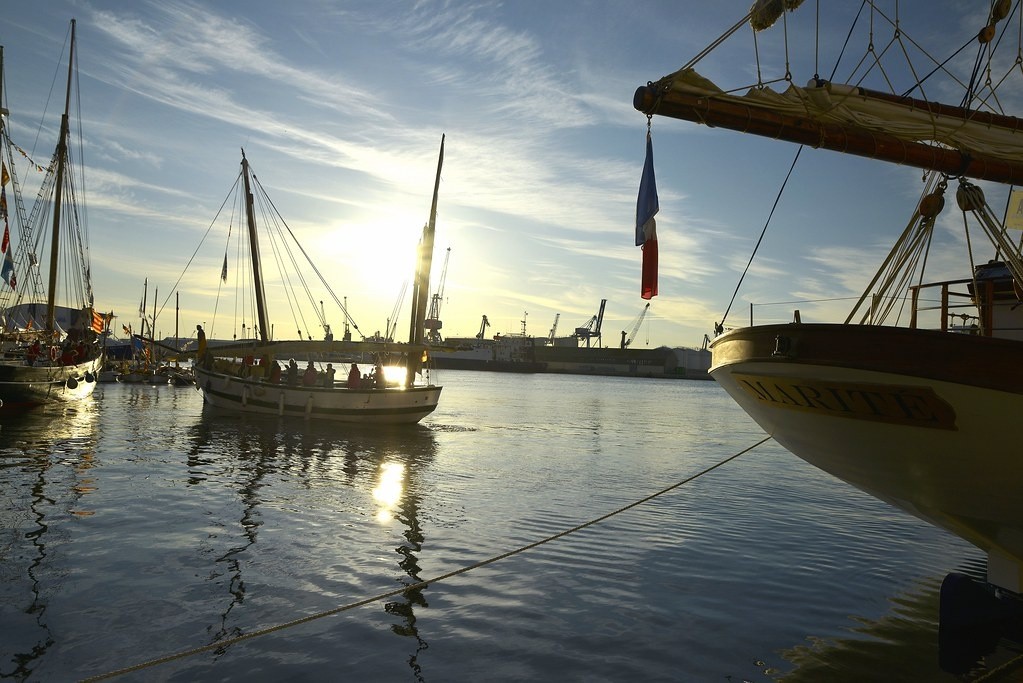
[95,278,196,384]
[0,17,109,409]
[634,0,1023,595]
[121,130,445,429]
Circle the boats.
[370,311,548,373]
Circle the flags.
[0,162,17,291]
[26,319,33,329]
[221,253,227,284]
[91,310,103,334]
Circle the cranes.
[341,296,351,342]
[320,300,333,341]
[545,313,559,346]
[621,301,651,348]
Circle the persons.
[323,364,334,388]
[197,325,207,364]
[302,360,318,387]
[347,364,360,388]
[271,360,281,384]
[373,368,386,389]
[285,358,298,385]
